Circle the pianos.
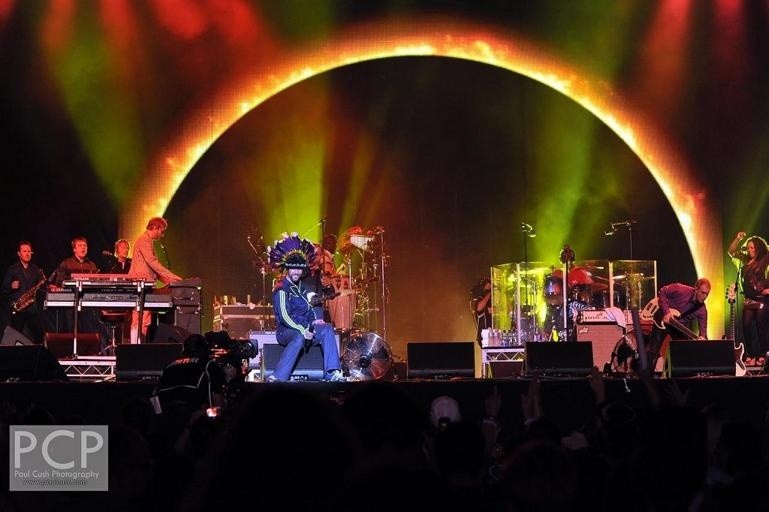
[63,278,154,291]
[163,277,202,289]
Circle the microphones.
[604,230,615,236]
[102,250,113,256]
[528,229,536,238]
[621,334,640,359]
[737,246,747,251]
[319,216,329,223]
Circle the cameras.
[205,406,224,418]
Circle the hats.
[285,254,307,269]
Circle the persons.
[1,239,46,344]
[337,220,365,275]
[726,230,769,367]
[146,334,769,511]
[475,266,514,349]
[269,250,349,381]
[47,235,100,334]
[641,278,712,380]
[364,226,394,337]
[314,231,354,289]
[99,237,132,356]
[129,217,185,343]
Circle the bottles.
[214,295,237,306]
[246,294,252,304]
[489,330,549,347]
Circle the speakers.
[663,339,736,379]
[576,324,671,372]
[0,344,69,382]
[524,341,593,378]
[406,341,475,379]
[0,326,35,346]
[261,344,324,381]
[44,332,101,355]
[116,343,184,382]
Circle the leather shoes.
[744,357,755,366]
[756,357,765,365]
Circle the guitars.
[722,284,747,377]
[640,297,697,340]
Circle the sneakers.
[324,370,346,382]
[266,375,284,383]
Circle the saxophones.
[13,274,46,311]
